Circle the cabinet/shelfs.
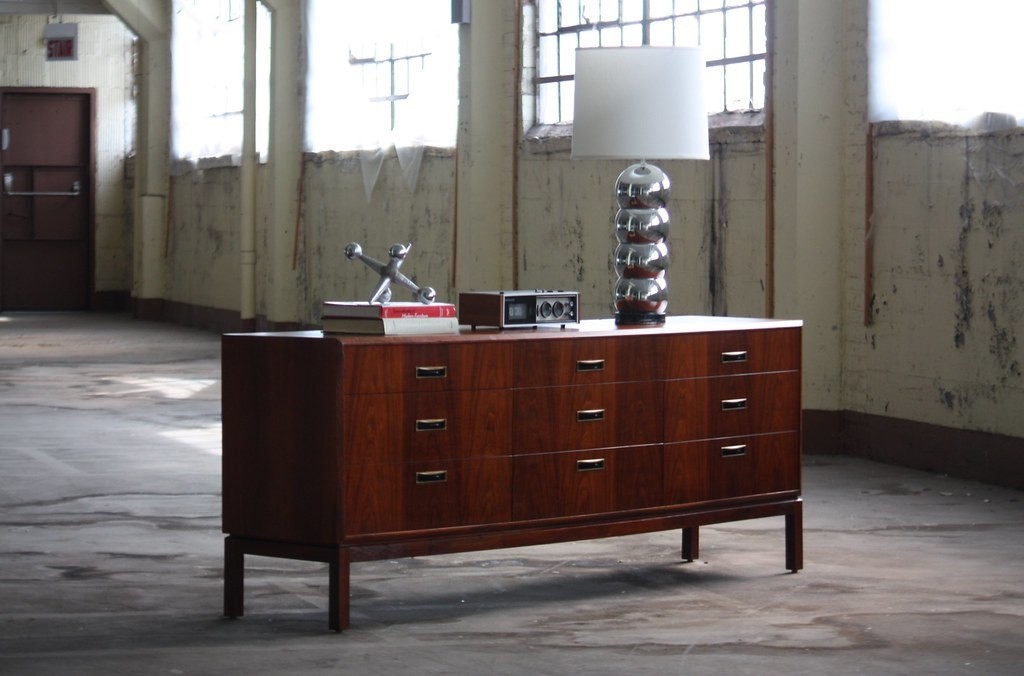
[220,313,802,635]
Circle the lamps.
[570,46,709,328]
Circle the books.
[321,301,458,336]
